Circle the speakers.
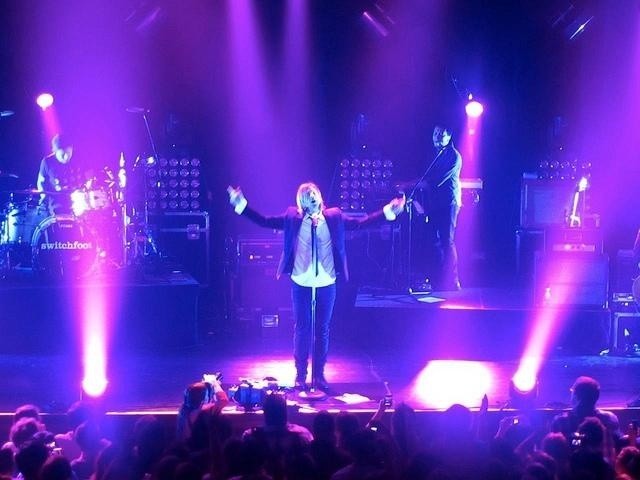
[229,236,295,330]
[533,250,609,309]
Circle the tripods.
[373,157,432,301]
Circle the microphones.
[311,213,319,228]
[126,105,151,113]
[436,142,448,152]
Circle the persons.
[411,121,462,292]
[36,133,97,216]
[1,372,639,480]
[226,182,406,390]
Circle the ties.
[309,216,318,276]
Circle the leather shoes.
[312,377,330,393]
[295,377,308,391]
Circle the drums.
[31,215,97,280]
[6,202,50,243]
[69,185,110,223]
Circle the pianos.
[395,179,484,189]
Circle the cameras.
[510,416,520,425]
[383,393,393,408]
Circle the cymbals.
[0,170,20,177]
[4,189,62,196]
